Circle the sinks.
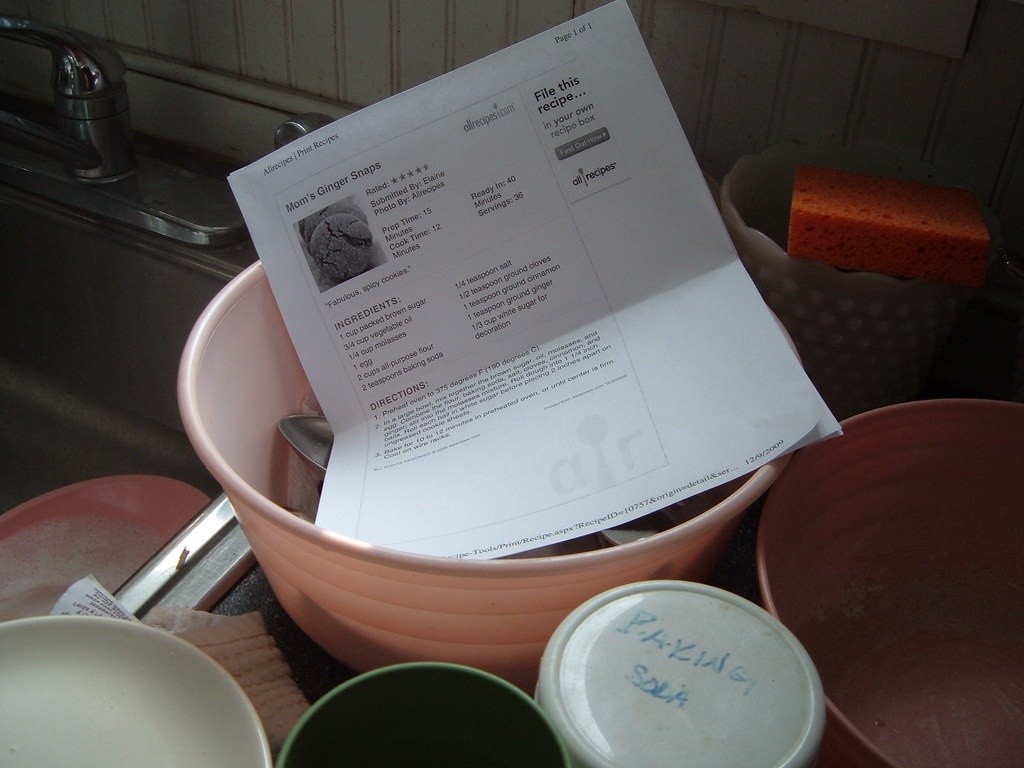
[0,182,257,624]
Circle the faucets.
[0,17,146,184]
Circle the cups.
[273,663,570,768]
[534,580,826,768]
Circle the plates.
[0,615,271,768]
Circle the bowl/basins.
[757,397,1024,768]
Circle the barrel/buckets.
[175,258,799,693]
[717,143,996,421]
